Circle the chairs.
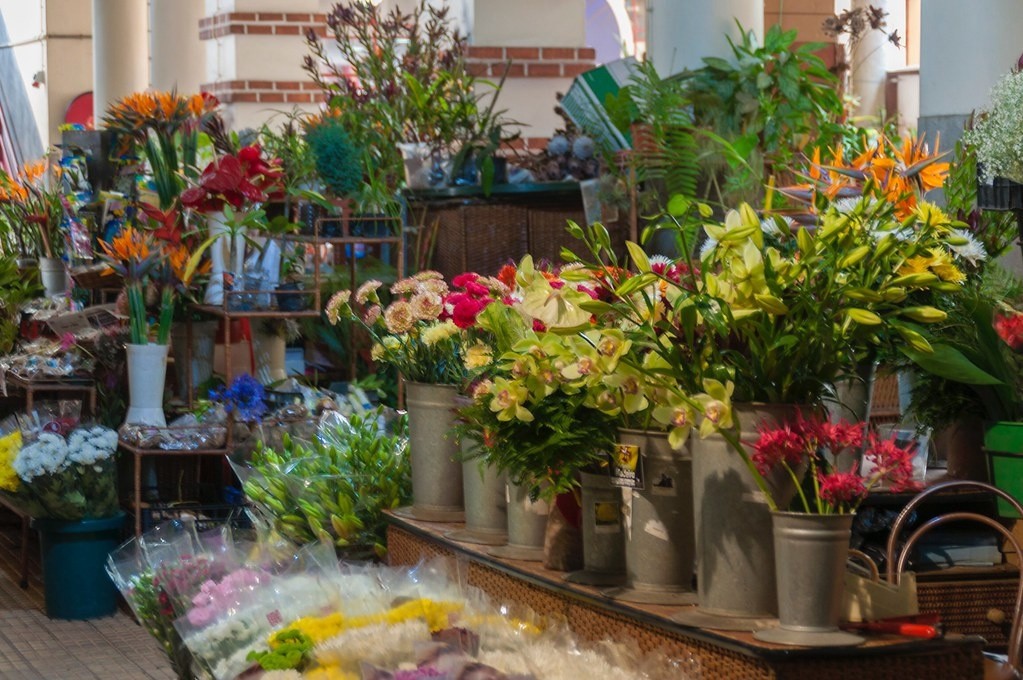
[877,550,1018,646]
[884,477,1023,680]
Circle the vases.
[398,376,465,522]
[299,201,326,238]
[504,449,551,556]
[577,461,621,574]
[444,391,508,547]
[124,337,170,439]
[765,507,857,629]
[201,206,242,304]
[39,253,66,297]
[684,398,812,620]
[612,419,696,591]
[268,197,294,233]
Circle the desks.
[370,498,987,680]
[398,178,628,275]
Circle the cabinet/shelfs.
[113,197,409,552]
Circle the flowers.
[0,0,1023,680]
